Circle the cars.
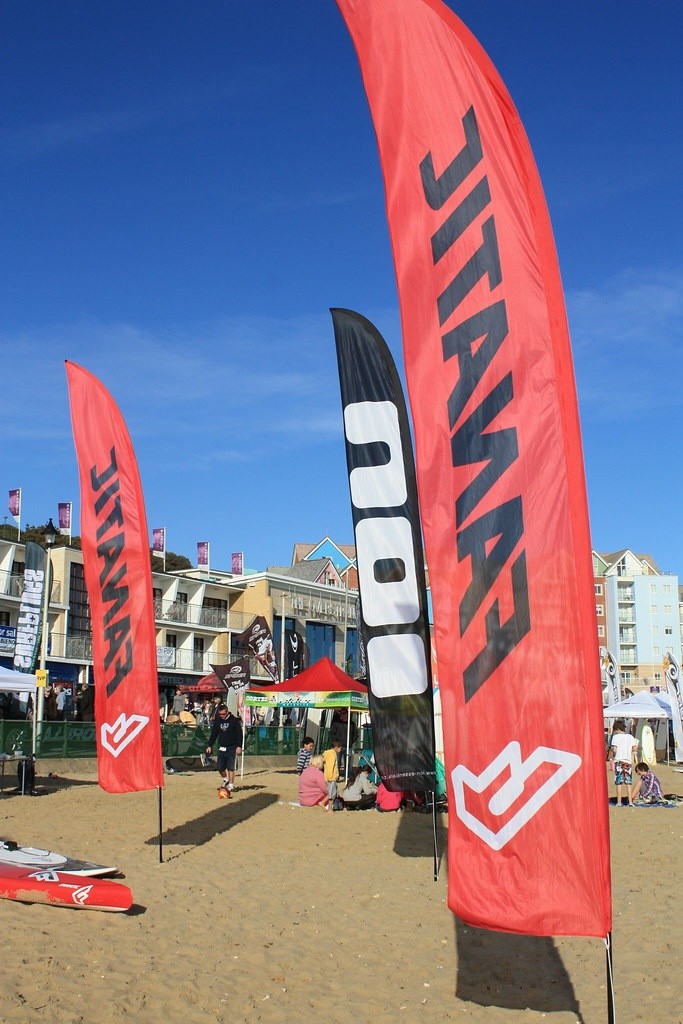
[0,702,35,756]
[28,694,82,720]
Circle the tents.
[0,666,38,764]
[603,691,674,766]
[241,657,370,783]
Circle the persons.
[331,708,357,778]
[206,705,243,791]
[631,763,663,801]
[342,765,376,810]
[321,741,343,799]
[376,779,422,813]
[296,736,314,776]
[159,690,223,726]
[298,755,328,810]
[1,680,93,724]
[610,721,636,807]
[251,719,300,752]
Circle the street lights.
[276,592,288,755]
[36,517,60,755]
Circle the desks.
[0,757,29,796]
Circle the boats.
[1,862,134,913]
[0,840,122,877]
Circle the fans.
[6,729,25,757]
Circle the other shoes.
[217,780,229,791]
[228,786,237,792]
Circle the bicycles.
[161,723,180,757]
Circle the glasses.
[220,712,227,715]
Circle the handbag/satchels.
[332,795,344,811]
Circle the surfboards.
[0,839,119,877]
[0,861,133,912]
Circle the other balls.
[218,788,231,799]
